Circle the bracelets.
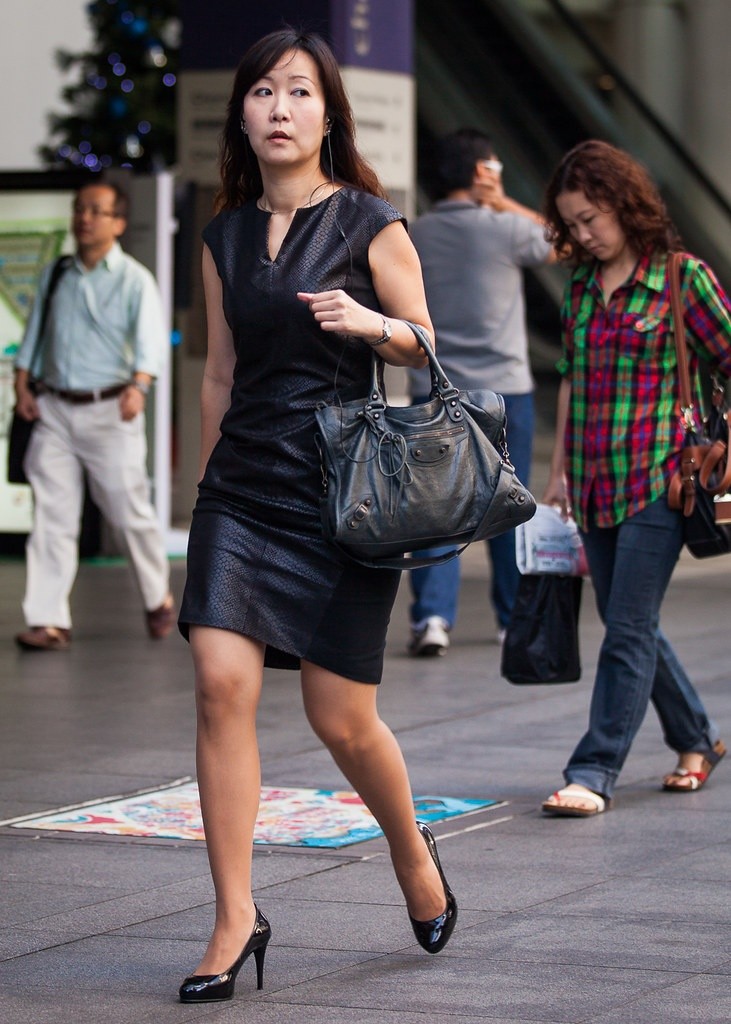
[134,381,150,394]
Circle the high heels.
[178,903,271,1004]
[399,821,458,955]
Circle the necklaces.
[259,182,330,214]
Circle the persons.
[178,29,458,1004]
[407,128,573,656]
[12,180,176,650]
[541,140,731,815]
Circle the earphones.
[326,117,332,134]
[241,115,246,130]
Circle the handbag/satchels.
[307,317,537,571]
[497,503,581,685]
[7,383,41,485]
[668,386,731,558]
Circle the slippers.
[542,788,608,816]
[660,737,724,791]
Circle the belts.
[49,386,124,404]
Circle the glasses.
[74,206,116,219]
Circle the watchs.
[371,314,392,346]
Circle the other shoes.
[15,626,70,650]
[146,593,176,639]
[407,616,451,657]
